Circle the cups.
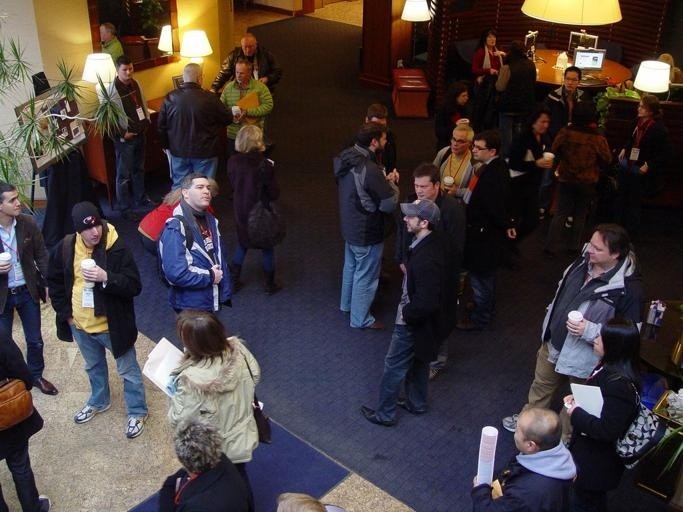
[231,105,241,116]
[542,152,555,161]
[567,311,583,322]
[80,259,96,288]
[443,175,453,187]
[0,252,12,265]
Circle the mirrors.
[87,0,181,72]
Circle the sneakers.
[74,402,111,423]
[139,196,152,205]
[503,415,517,433]
[125,412,148,438]
[455,319,487,331]
[368,321,385,328]
[36,495,51,512]
[122,210,136,221]
[428,366,447,380]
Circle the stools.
[119,34,145,62]
[390,68,429,119]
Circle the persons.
[47,202,148,438]
[1,23,283,296]
[156,173,233,316]
[277,493,327,511]
[1,183,58,395]
[333,30,683,511]
[159,417,248,511]
[167,307,260,511]
[1,332,51,511]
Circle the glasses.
[471,144,491,151]
[451,136,466,144]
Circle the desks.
[83,87,230,211]
[525,48,632,90]
[639,297,682,379]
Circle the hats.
[72,201,102,233]
[401,200,441,228]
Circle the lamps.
[82,52,118,104]
[179,29,214,74]
[157,24,172,54]
[633,60,671,99]
[400,0,432,68]
[521,0,623,27]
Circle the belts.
[7,285,27,296]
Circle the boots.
[230,265,242,293]
[263,269,282,294]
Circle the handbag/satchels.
[617,402,670,469]
[248,202,286,250]
[253,408,270,443]
[0,379,33,432]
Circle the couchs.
[450,26,522,81]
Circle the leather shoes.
[360,405,380,424]
[32,378,58,394]
[397,397,425,413]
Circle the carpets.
[128,416,352,511]
[20,15,683,511]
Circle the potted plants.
[140,0,166,61]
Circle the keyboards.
[580,79,608,85]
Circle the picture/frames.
[14,81,90,175]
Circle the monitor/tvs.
[525,31,539,63]
[568,31,599,52]
[573,48,606,71]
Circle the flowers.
[623,386,683,484]
